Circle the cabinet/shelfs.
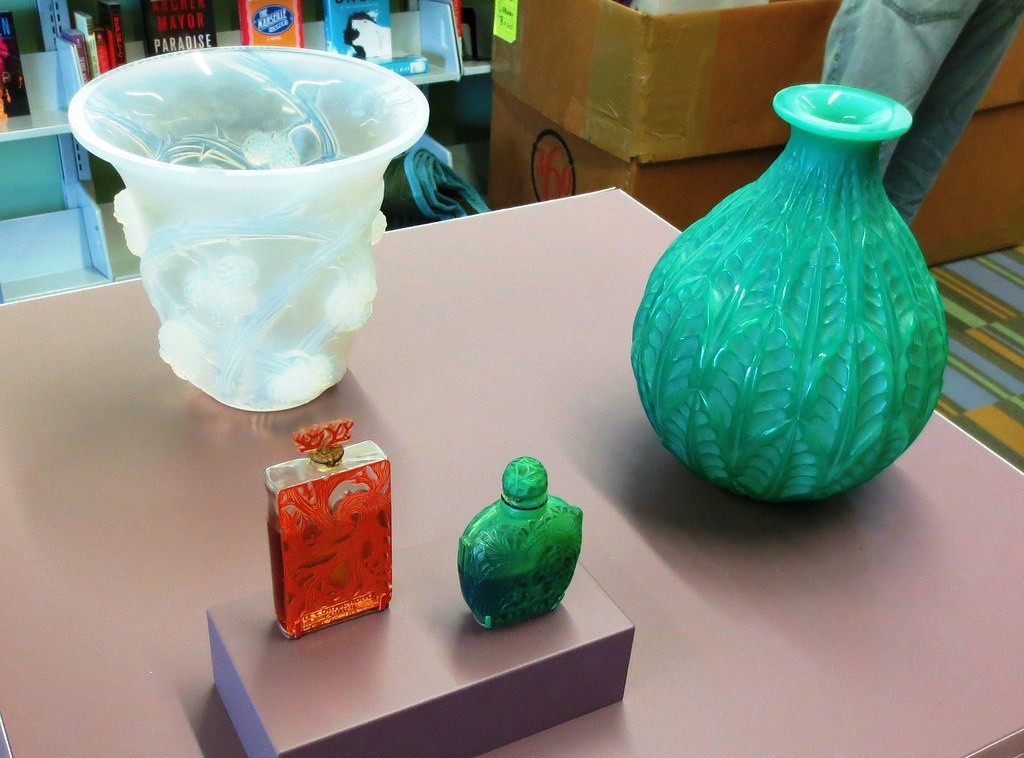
[0,0,493,305]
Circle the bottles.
[264,417,392,638]
[630,84,949,505]
[457,456,583,629]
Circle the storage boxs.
[495,0,1024,166]
[490,84,1024,265]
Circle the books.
[139,0,218,59]
[378,55,429,76]
[322,0,393,65]
[0,9,30,122]
[445,0,464,60]
[61,0,127,85]
[237,0,304,49]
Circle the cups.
[66,45,431,414]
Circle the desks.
[0,186,1023,758]
